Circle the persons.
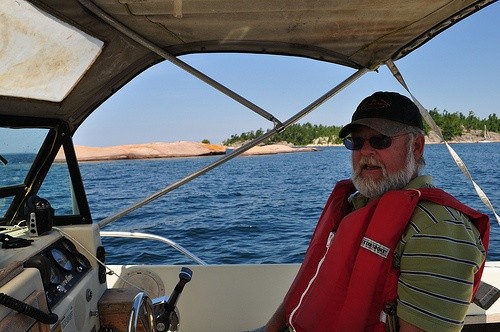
[253,91,490,332]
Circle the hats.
[339,91,423,137]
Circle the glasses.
[341,132,412,151]
